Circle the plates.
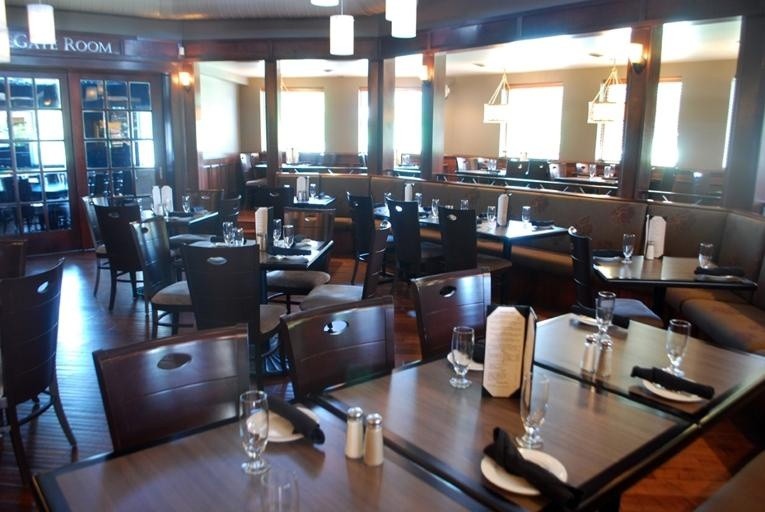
[245,405,321,443]
[577,313,613,326]
[641,377,703,403]
[479,446,568,496]
[447,348,484,371]
[594,256,621,262]
[703,275,737,281]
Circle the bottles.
[365,414,384,466]
[580,335,597,376]
[344,407,365,459]
[598,340,614,377]
[647,241,654,261]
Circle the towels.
[268,395,326,445]
[483,427,581,512]
[694,266,746,278]
[630,365,715,400]
[423,206,431,211]
[295,234,306,242]
[486,170,500,173]
[399,164,415,167]
[592,249,632,259]
[290,162,303,165]
[532,219,555,226]
[571,301,629,329]
[319,192,325,199]
[440,341,485,363]
[479,212,487,217]
[266,246,311,256]
[168,212,194,218]
[210,237,224,243]
[419,212,429,216]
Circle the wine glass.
[609,164,616,179]
[516,374,553,448]
[239,390,273,475]
[699,242,714,269]
[521,206,531,227]
[449,326,475,390]
[592,291,616,342]
[259,467,299,512]
[661,319,691,378]
[431,198,440,220]
[589,163,597,182]
[181,193,192,214]
[487,206,496,234]
[283,225,295,249]
[232,227,245,248]
[272,218,282,247]
[309,183,316,201]
[621,233,636,264]
[223,222,234,247]
[383,191,392,214]
[414,193,422,212]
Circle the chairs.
[346,192,374,286]
[92,323,250,456]
[279,293,397,396]
[576,163,591,175]
[251,151,262,178]
[255,207,331,319]
[568,226,663,326]
[129,215,194,340]
[412,267,493,357]
[191,195,242,234]
[437,208,512,268]
[547,162,566,177]
[239,153,268,204]
[386,196,441,300]
[94,204,149,311]
[1,239,29,277]
[81,191,140,299]
[457,158,496,171]
[651,166,724,195]
[86,169,125,207]
[531,161,550,181]
[300,227,388,310]
[5,177,46,233]
[21,175,63,184]
[506,161,528,177]
[179,242,288,391]
[0,257,78,487]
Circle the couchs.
[278,172,370,256]
[373,177,644,290]
[663,212,763,358]
[644,202,765,363]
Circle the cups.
[604,166,611,179]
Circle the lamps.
[629,53,645,71]
[27,1,58,44]
[86,86,98,102]
[418,67,431,85]
[179,69,192,91]
[483,80,522,123]
[329,0,355,55]
[391,0,417,38]
[587,68,626,122]
